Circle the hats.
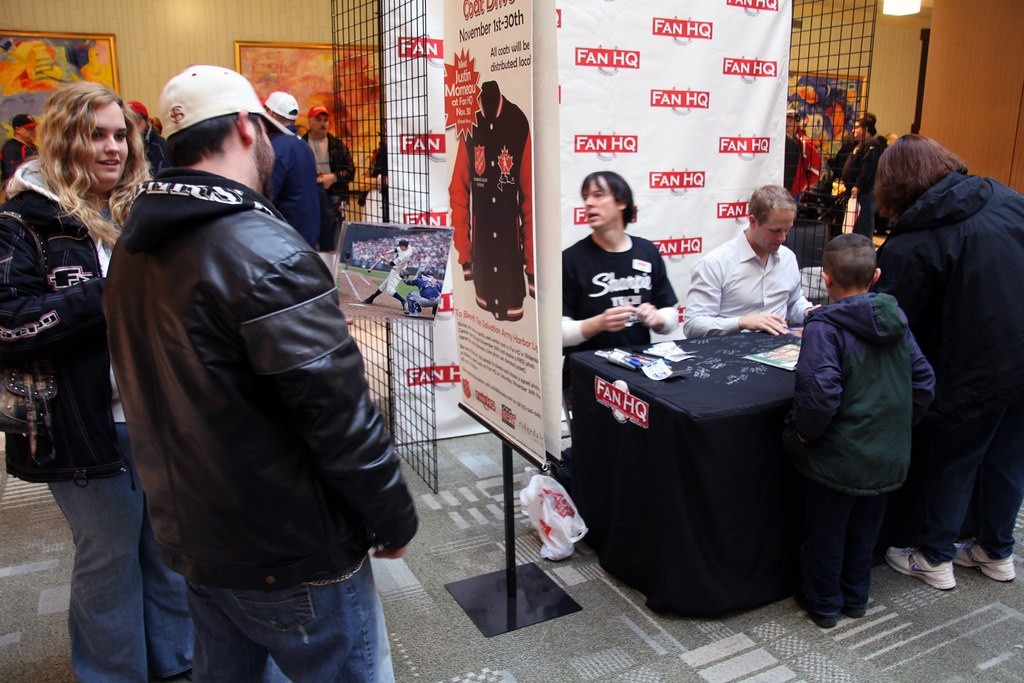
[786,109,801,118]
[264,90,299,121]
[126,101,148,119]
[308,106,329,121]
[12,114,39,128]
[159,65,295,141]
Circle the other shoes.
[792,593,865,627]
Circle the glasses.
[852,123,862,129]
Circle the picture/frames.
[233,41,381,226]
[0,29,121,152]
[786,71,866,196]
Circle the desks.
[561,324,916,620]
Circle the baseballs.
[362,265,366,268]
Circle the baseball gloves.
[399,270,409,278]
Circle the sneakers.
[885,545,956,589]
[953,539,1015,580]
[403,303,419,317]
[362,297,373,304]
[540,542,576,561]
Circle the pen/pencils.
[594,348,670,371]
[782,324,787,328]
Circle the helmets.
[399,239,408,246]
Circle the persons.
[786,109,821,188]
[301,104,354,251]
[824,138,857,179]
[0,114,41,187]
[126,102,168,178]
[843,120,881,240]
[401,270,443,318]
[868,134,1024,589]
[264,92,322,250]
[102,63,418,683]
[782,233,935,626]
[783,136,800,193]
[562,170,683,462]
[682,184,822,337]
[862,114,888,150]
[366,120,389,221]
[0,83,196,683]
[362,238,413,310]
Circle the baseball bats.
[368,258,382,273]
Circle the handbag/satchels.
[520,475,587,557]
[0,211,60,436]
